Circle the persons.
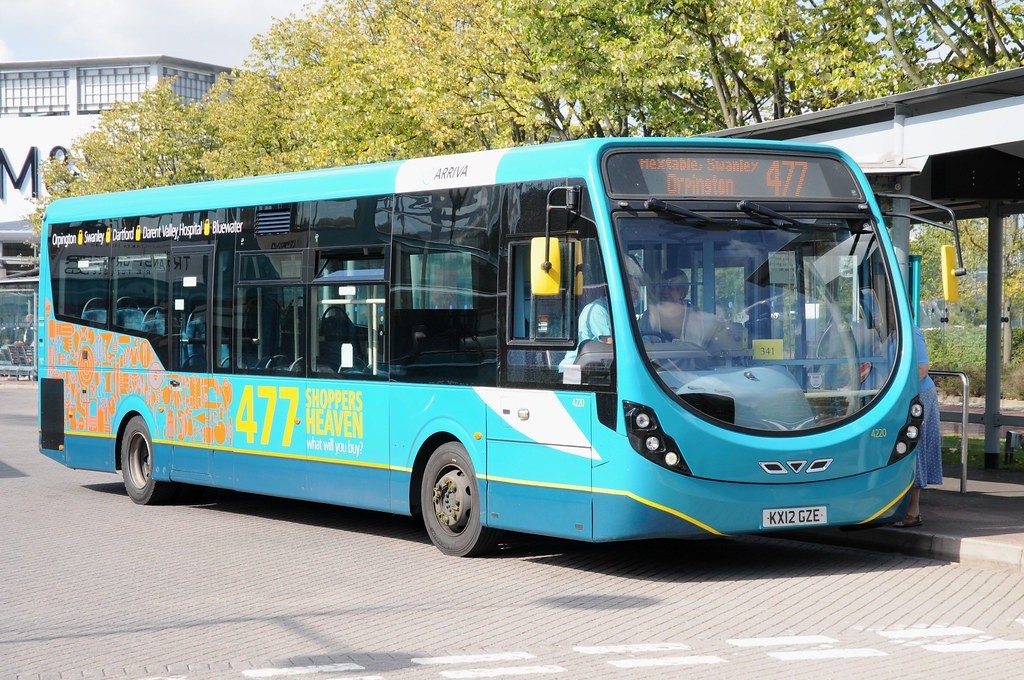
[558,274,655,373]
[880,295,943,527]
[0,314,34,363]
[649,269,701,337]
[226,254,279,358]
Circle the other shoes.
[894,513,924,528]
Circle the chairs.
[80,297,369,376]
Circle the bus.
[34,134,969,559]
